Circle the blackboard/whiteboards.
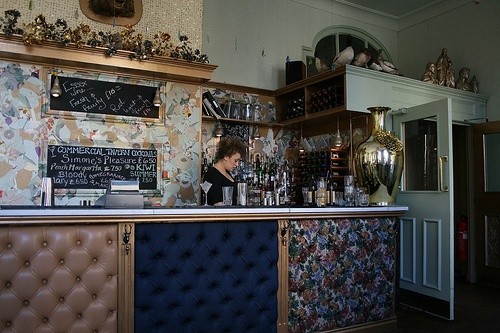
[50,74,160,118]
[47,144,157,190]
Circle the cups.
[237,183,248,207]
[222,186,233,206]
[40,177,55,207]
[335,174,370,206]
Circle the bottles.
[231,150,296,206]
[202,147,215,179]
[224,93,276,122]
[285,85,340,119]
[326,174,336,206]
[316,177,327,207]
[308,173,316,207]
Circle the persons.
[422,48,479,93]
[203,137,247,207]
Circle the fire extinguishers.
[457,214,468,259]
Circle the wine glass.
[200,184,212,206]
[302,186,310,207]
